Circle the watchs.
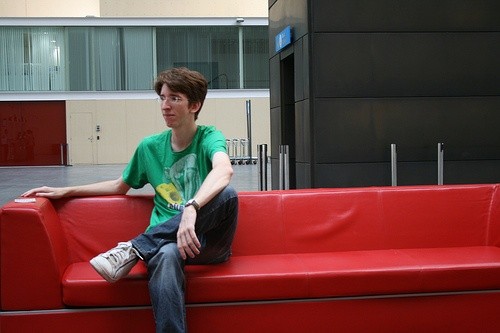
[184,198,200,213]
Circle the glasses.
[156,95,189,104]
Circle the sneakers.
[90,240,144,283]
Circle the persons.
[20,66,239,333]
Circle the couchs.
[0,182,500,333]
